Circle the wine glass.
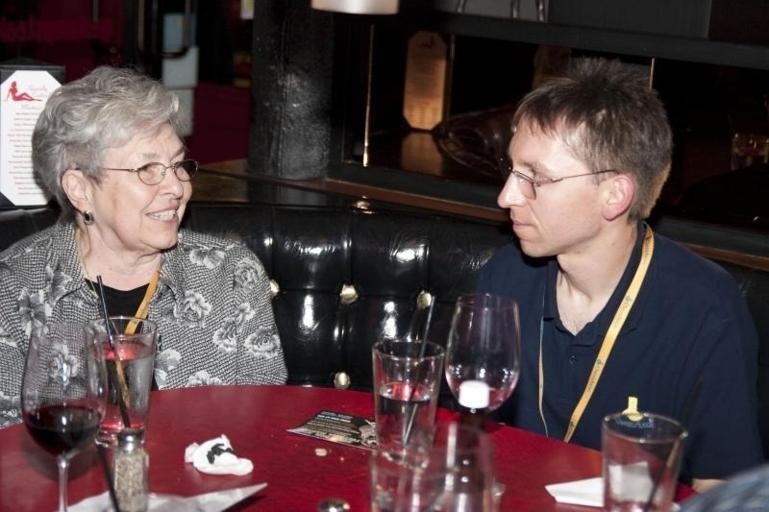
[441,291,522,415]
[21,321,109,511]
[391,427,495,509]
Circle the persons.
[0,65,288,426]
[464,54,767,492]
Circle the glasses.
[500,158,619,200]
[104,159,198,186]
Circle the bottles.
[112,429,148,511]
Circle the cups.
[368,446,446,510]
[733,129,769,230]
[81,314,157,449]
[371,341,444,454]
[602,416,687,512]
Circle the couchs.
[431,105,682,214]
[0,206,769,459]
